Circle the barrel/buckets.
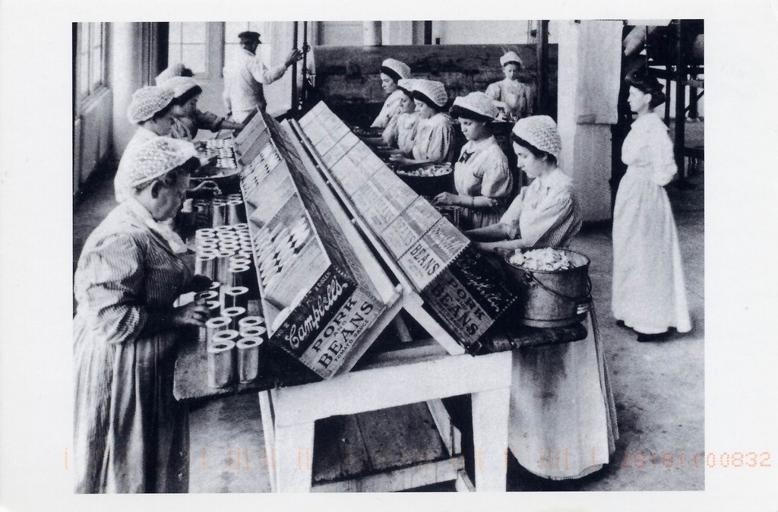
[506,246,593,330]
[396,162,455,199]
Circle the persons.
[371,57,410,128]
[219,31,305,123]
[71,134,209,495]
[155,65,244,140]
[163,79,218,177]
[429,90,515,245]
[116,87,220,228]
[455,113,623,485]
[378,80,452,170]
[383,79,418,148]
[609,65,697,341]
[486,50,533,122]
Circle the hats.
[239,32,262,44]
[130,137,197,187]
[512,115,562,158]
[453,91,499,119]
[127,63,196,125]
[382,58,448,107]
[500,51,522,67]
